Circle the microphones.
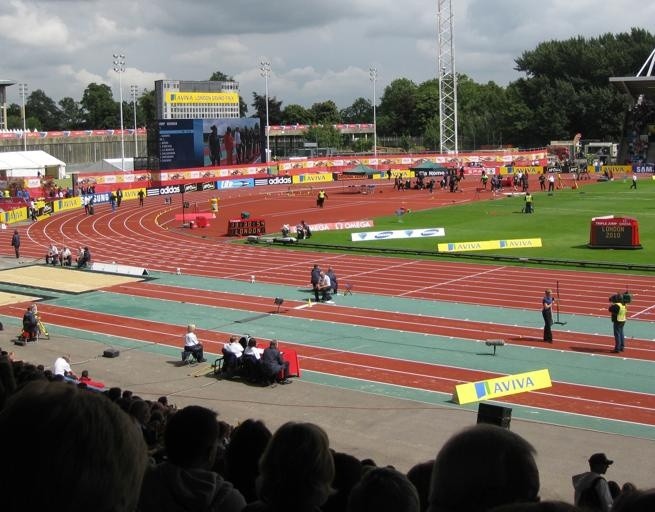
[552,298,554,301]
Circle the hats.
[187,324,195,332]
[588,453,614,466]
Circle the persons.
[387,167,391,179]
[182,324,205,362]
[460,166,465,179]
[224,334,292,384]
[0,175,96,223]
[0,350,655,512]
[137,190,146,205]
[209,121,261,164]
[479,166,555,214]
[44,242,92,269]
[9,230,22,261]
[543,287,557,343]
[595,160,603,172]
[318,187,328,208]
[23,304,48,341]
[110,186,122,212]
[609,293,628,353]
[630,173,638,189]
[560,152,570,165]
[395,172,460,193]
[310,263,339,302]
[285,171,289,174]
[300,221,311,238]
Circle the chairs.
[221,346,241,380]
[258,357,286,388]
[240,354,265,385]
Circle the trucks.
[367,144,401,153]
[549,140,619,165]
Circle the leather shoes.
[282,380,292,384]
[200,358,208,362]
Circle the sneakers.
[609,349,619,353]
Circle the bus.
[297,148,337,158]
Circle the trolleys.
[295,225,311,239]
[521,202,536,213]
[571,175,580,192]
[475,181,486,192]
[554,173,565,193]
[315,198,324,207]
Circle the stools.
[20,330,39,343]
[323,288,334,302]
[343,283,356,296]
[181,350,198,364]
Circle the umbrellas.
[411,161,447,174]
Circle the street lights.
[112,53,127,171]
[369,61,377,155]
[18,82,29,151]
[259,59,274,159]
[573,133,582,160]
[130,83,140,155]
[178,183,187,225]
